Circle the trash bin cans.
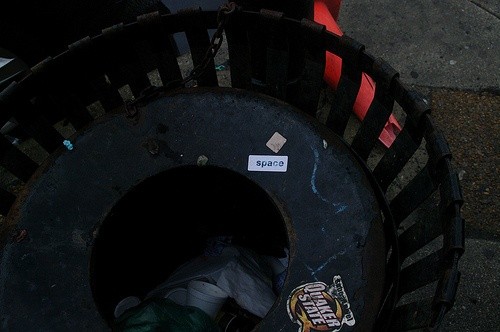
[1,7,466,332]
